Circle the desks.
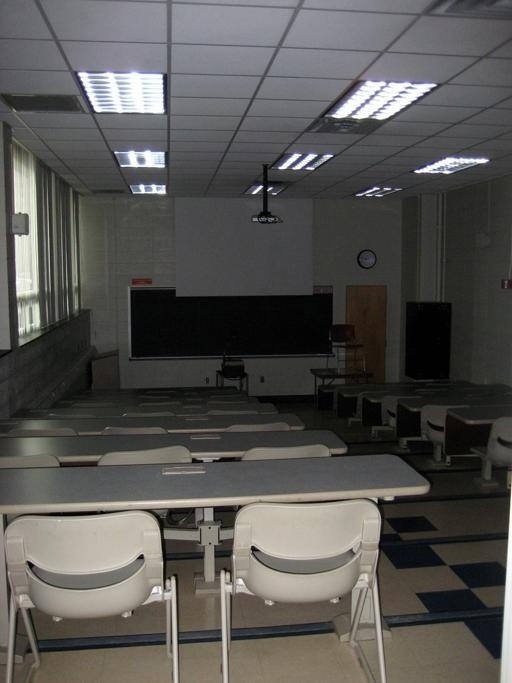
[54,386,277,414]
[0,429,349,462]
[0,455,431,664]
[397,395,512,452]
[317,380,454,412]
[361,383,511,431]
[332,381,477,425]
[311,368,371,411]
[443,406,512,468]
[1,411,305,431]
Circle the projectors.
[250,212,286,224]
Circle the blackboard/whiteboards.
[127,285,334,360]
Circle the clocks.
[357,249,377,270]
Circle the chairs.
[0,454,61,468]
[369,398,422,439]
[102,426,167,435]
[471,416,512,491]
[326,323,366,372]
[348,392,372,427]
[224,422,291,433]
[421,403,470,463]
[4,510,179,683]
[6,427,77,436]
[98,445,192,466]
[219,498,387,683]
[242,445,332,460]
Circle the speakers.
[405,300,453,381]
